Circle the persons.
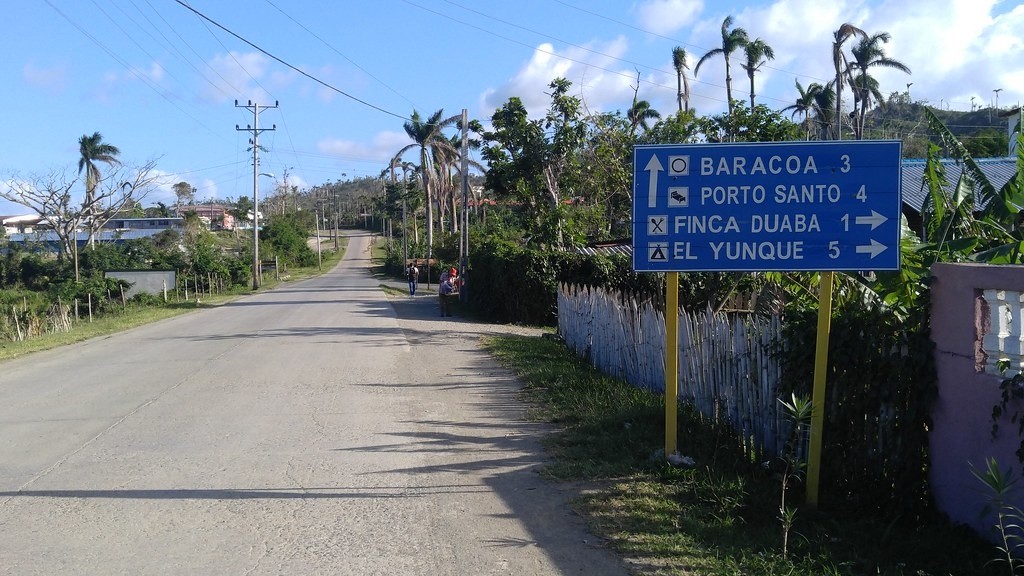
[439,266,465,317]
[406,263,420,295]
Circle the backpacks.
[409,267,415,281]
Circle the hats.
[450,267,457,277]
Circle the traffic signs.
[629,142,903,274]
